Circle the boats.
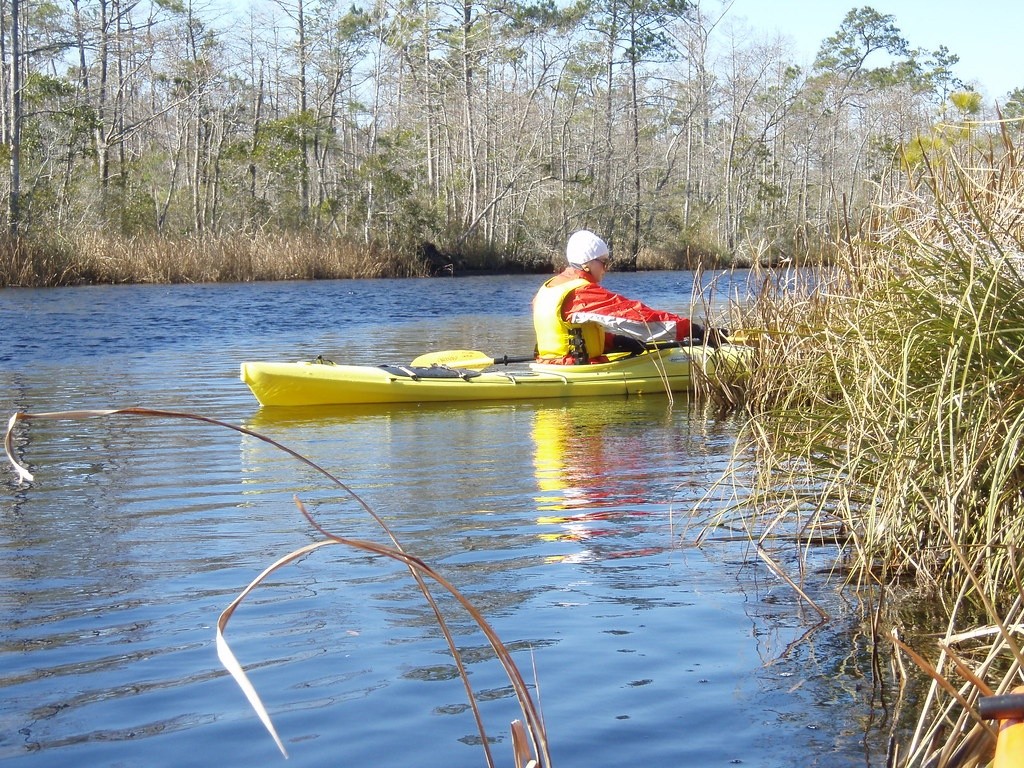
[237,344,777,407]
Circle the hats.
[567,230,608,264]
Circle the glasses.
[595,258,608,270]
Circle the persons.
[531,228,728,365]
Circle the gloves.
[614,334,646,356]
[692,324,730,348]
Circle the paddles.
[411,328,779,374]
